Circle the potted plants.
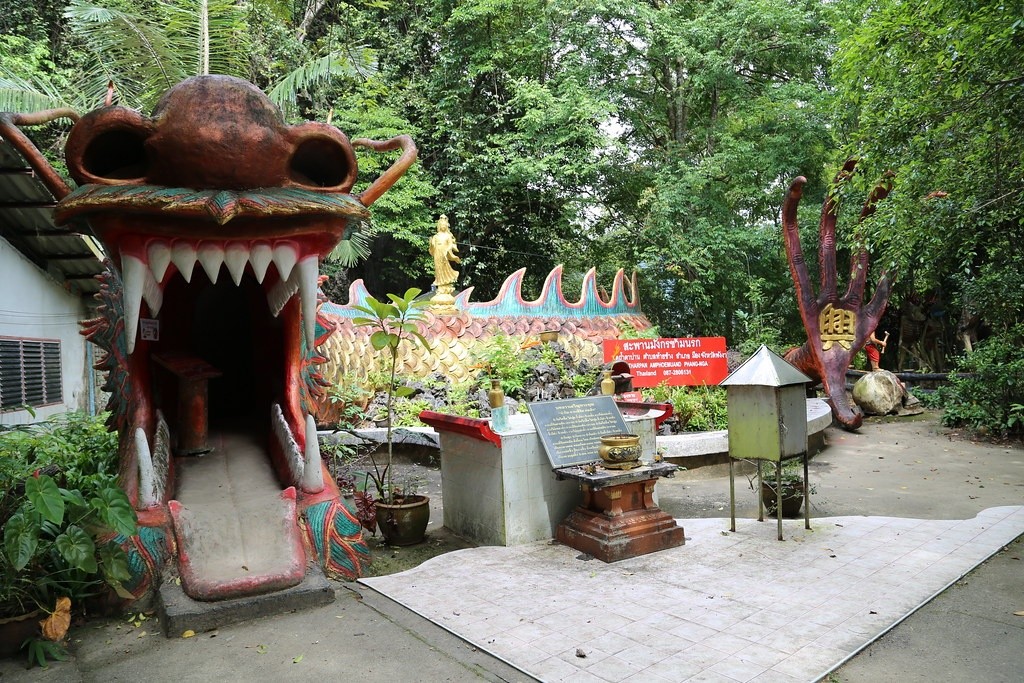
[0,440,135,670]
[762,458,816,517]
[318,288,435,548]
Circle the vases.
[489,379,503,408]
[601,371,615,396]
[540,331,560,344]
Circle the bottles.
[600,370,615,396]
[488,379,504,408]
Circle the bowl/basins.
[539,331,560,343]
